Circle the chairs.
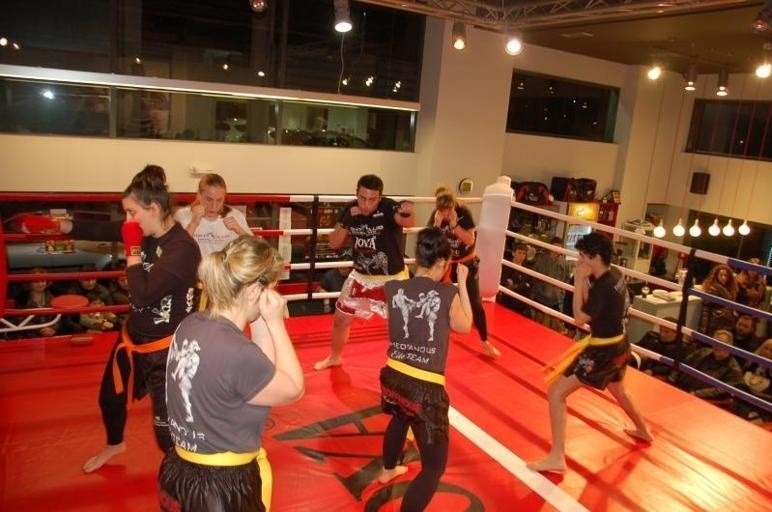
[642,361,771,431]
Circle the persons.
[427,187,503,358]
[380,226,473,511]
[314,174,417,370]
[317,254,353,312]
[158,235,306,512]
[174,173,255,313]
[81,164,203,472]
[8,259,131,339]
[527,230,653,471]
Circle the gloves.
[123,218,144,256]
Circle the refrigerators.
[562,201,599,277]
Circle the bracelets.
[450,224,458,230]
[399,212,412,218]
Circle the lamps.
[649,3,771,242]
[249,1,525,57]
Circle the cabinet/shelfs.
[621,218,656,278]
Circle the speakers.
[689,171,710,195]
